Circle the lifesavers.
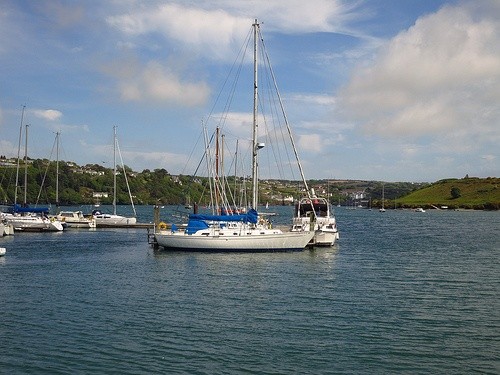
[158,222,166,229]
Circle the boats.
[415,206,449,212]
[290,188,340,247]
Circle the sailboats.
[0,105,96,237]
[148,19,322,248]
[84,125,137,227]
[379,184,386,212]
